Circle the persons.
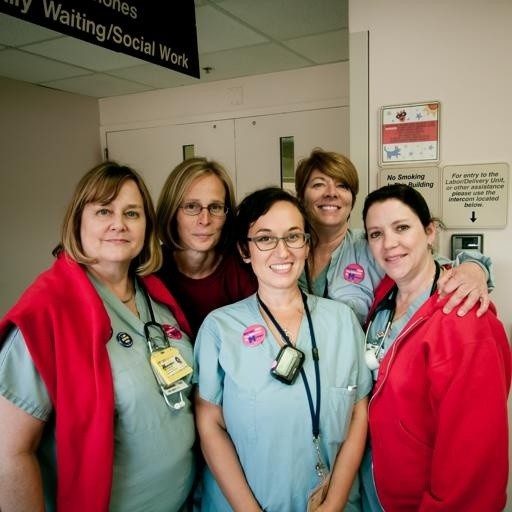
[0,162,196,512]
[360,185,512,512]
[191,186,373,511]
[295,146,495,327]
[152,157,259,347]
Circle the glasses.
[177,202,229,216]
[245,232,311,252]
